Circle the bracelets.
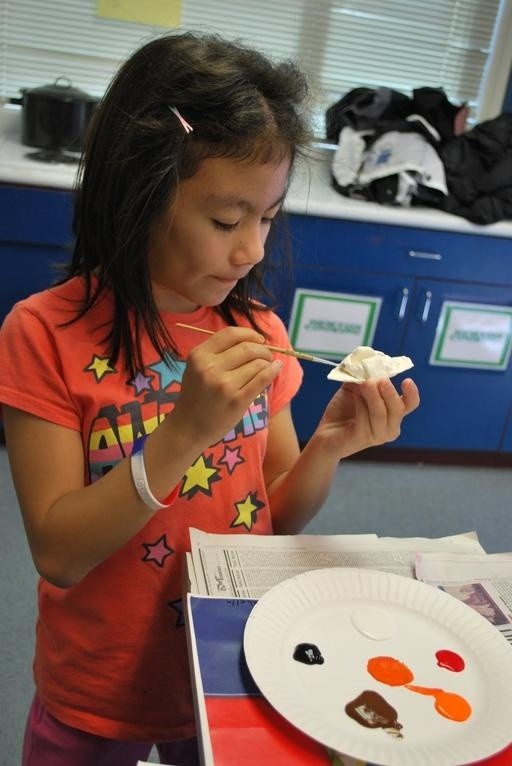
[130,432,184,510]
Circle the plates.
[243,566,512,765]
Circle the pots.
[0,76,99,154]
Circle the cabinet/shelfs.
[0,108,512,467]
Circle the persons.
[0,23,425,766]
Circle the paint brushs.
[177,322,344,366]
